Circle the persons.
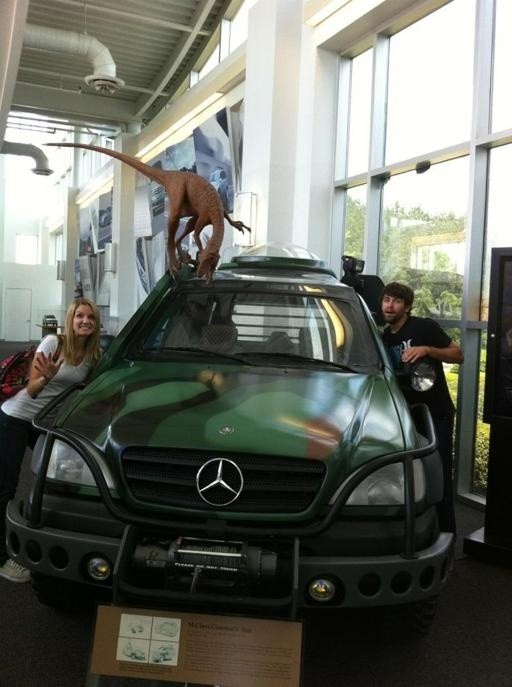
[378,282,465,583]
[0,297,101,584]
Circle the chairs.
[194,324,340,362]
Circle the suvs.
[13,248,464,637]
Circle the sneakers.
[0,559,32,583]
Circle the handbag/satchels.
[0,346,36,401]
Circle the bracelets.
[38,378,47,388]
